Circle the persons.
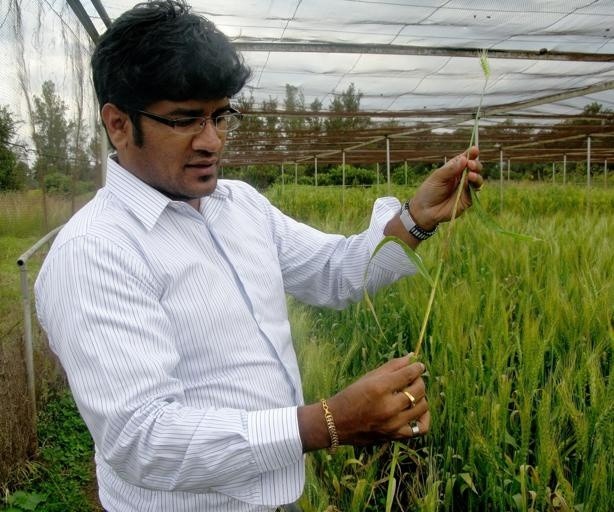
[30,1,485,512]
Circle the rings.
[410,421,421,439]
[399,389,417,408]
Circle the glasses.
[121,102,247,136]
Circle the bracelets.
[399,197,438,240]
[319,399,338,456]
